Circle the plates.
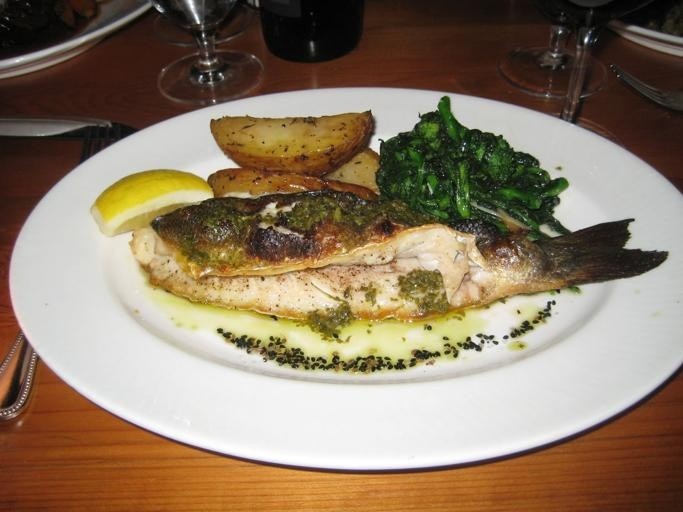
[10,86,681,473]
[2,0,152,83]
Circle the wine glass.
[146,0,266,107]
[497,0,654,142]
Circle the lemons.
[90,170,215,238]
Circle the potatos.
[206,110,385,202]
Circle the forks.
[0,120,118,422]
